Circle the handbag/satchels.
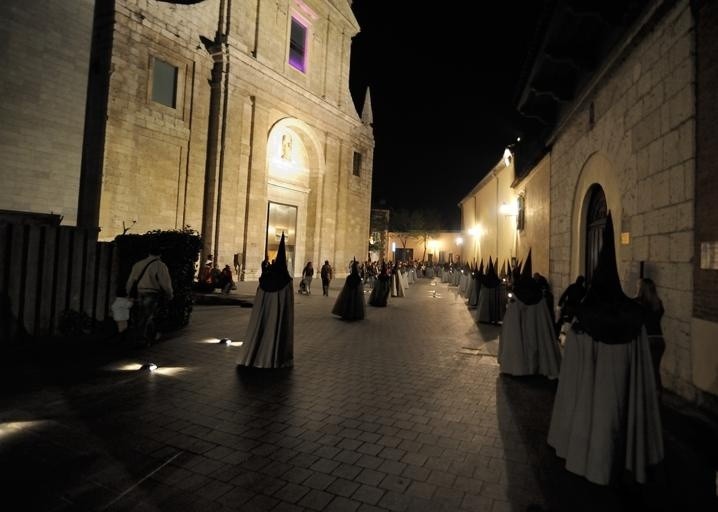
[130,279,138,299]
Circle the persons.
[320,261,332,296]
[302,262,314,295]
[349,257,467,289]
[199,254,234,296]
[633,277,668,395]
[125,242,174,350]
[552,275,585,341]
